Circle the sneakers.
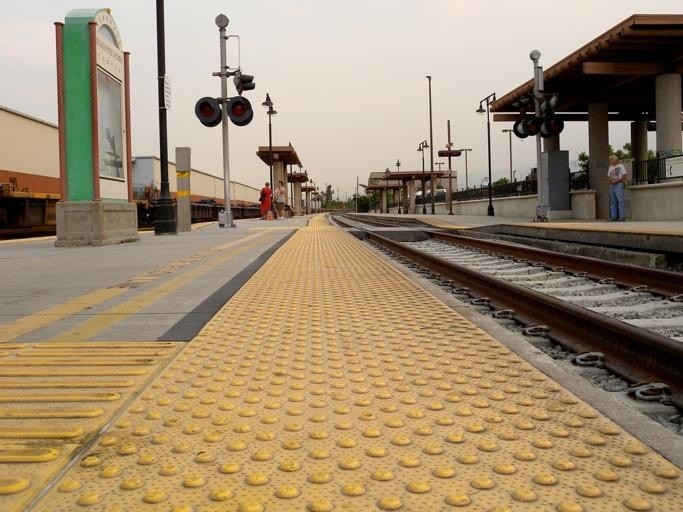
[606,218,624,222]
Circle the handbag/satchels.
[259,193,265,201]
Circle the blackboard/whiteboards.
[569,189,597,220]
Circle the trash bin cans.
[217,209,226,228]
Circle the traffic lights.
[194,94,253,128]
[511,116,563,140]
[232,69,255,96]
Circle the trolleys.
[531,205,550,224]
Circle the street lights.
[476,93,501,215]
[395,158,401,214]
[426,73,436,214]
[416,140,429,214]
[262,91,276,214]
[513,169,516,181]
[304,170,309,209]
[461,148,474,189]
[435,162,444,172]
[502,128,518,183]
[287,142,294,210]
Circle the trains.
[0,104,292,242]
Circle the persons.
[258,182,272,220]
[274,180,286,220]
[607,154,627,221]
